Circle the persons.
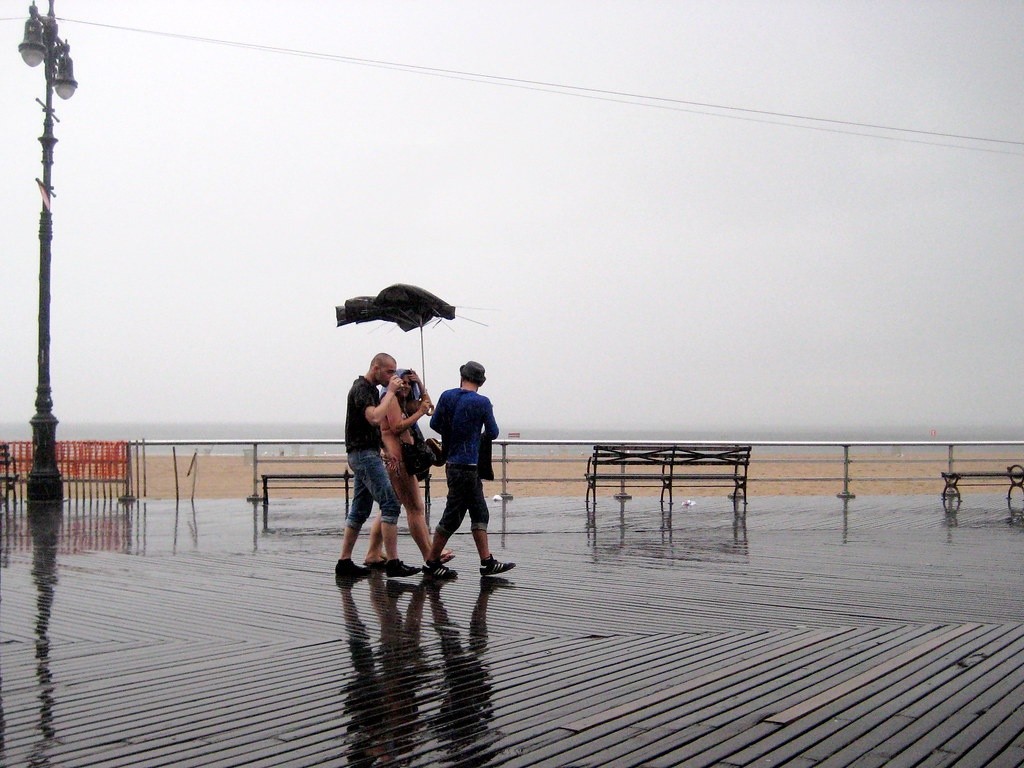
[423,361,516,577]
[335,353,422,577]
[363,368,455,569]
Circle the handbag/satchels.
[401,438,436,475]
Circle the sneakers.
[480,554,516,575]
[421,560,458,578]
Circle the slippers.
[440,553,455,563]
[363,559,387,568]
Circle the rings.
[397,380,400,383]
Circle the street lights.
[19,0,80,541]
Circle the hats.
[459,361,486,384]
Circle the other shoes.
[386,560,421,577]
[335,561,371,575]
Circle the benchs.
[941,464,1024,501]
[583,443,748,501]
[261,470,433,520]
[0,444,20,502]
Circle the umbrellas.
[336,285,491,416]
